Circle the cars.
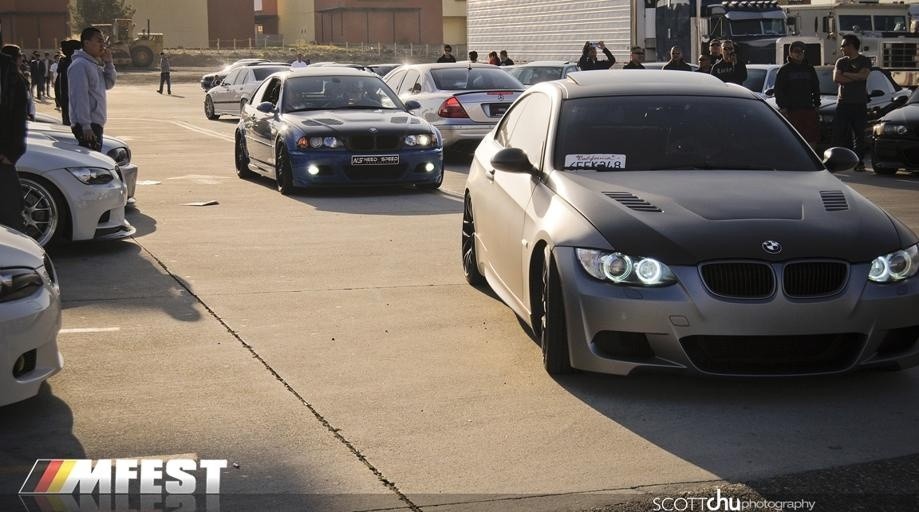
[451,69,918,385]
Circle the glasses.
[671,52,680,56]
[721,46,734,51]
[631,52,644,55]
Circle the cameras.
[589,41,600,48]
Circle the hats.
[697,53,713,61]
[709,39,721,45]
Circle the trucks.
[776,2,919,71]
[699,0,801,68]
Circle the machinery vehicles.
[77,17,169,72]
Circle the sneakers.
[854,163,866,172]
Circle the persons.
[468,51,481,63]
[437,44,457,63]
[0,25,120,234]
[487,50,500,66]
[155,52,172,95]
[579,34,873,175]
[291,54,307,67]
[496,51,514,66]
